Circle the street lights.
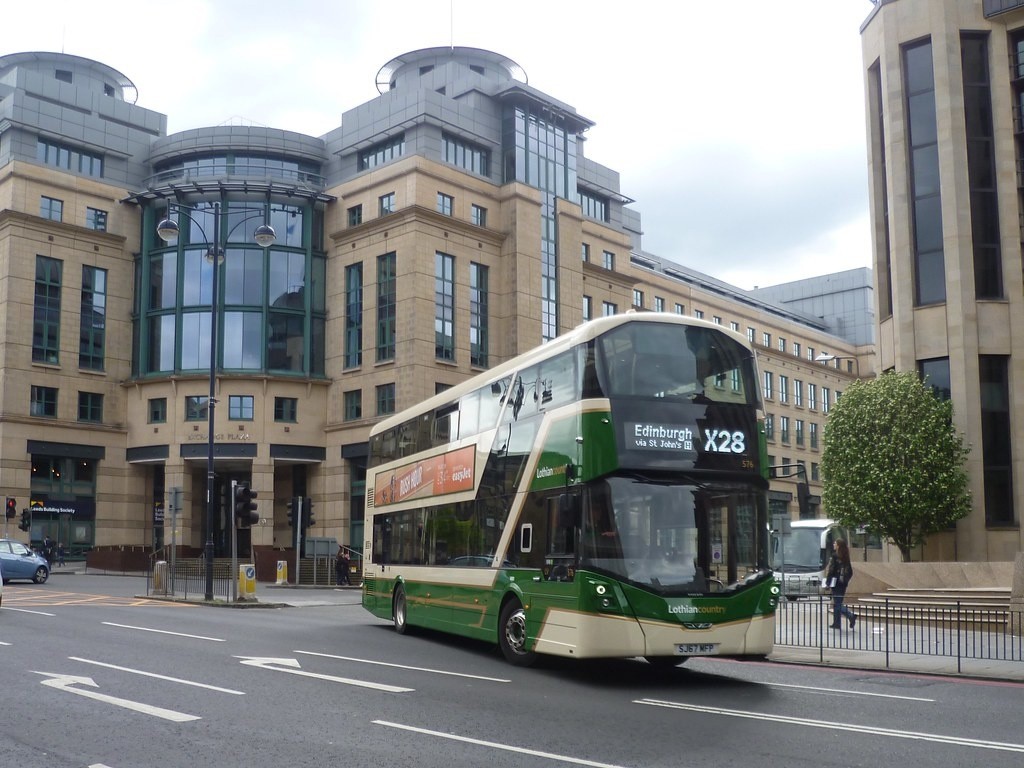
[155,197,276,599]
[815,355,860,380]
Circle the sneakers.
[849,614,857,628]
[829,624,841,629]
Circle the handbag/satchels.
[837,575,847,586]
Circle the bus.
[773,519,882,602]
[362,309,778,681]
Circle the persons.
[334,546,354,586]
[569,498,618,537]
[24,535,67,572]
[824,537,858,630]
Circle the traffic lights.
[234,485,250,527]
[243,482,260,526]
[19,509,31,532]
[5,497,16,519]
[286,497,299,528]
[301,498,316,527]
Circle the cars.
[0,538,50,584]
